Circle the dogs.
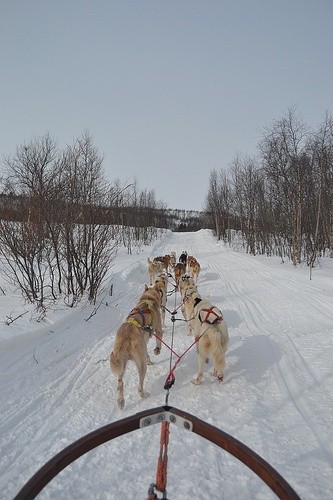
[179,251,188,268]
[152,277,168,327]
[190,293,229,385]
[110,282,162,411]
[188,256,200,283]
[147,257,164,285]
[153,255,171,274]
[179,278,201,336]
[174,263,186,285]
[169,251,176,272]
